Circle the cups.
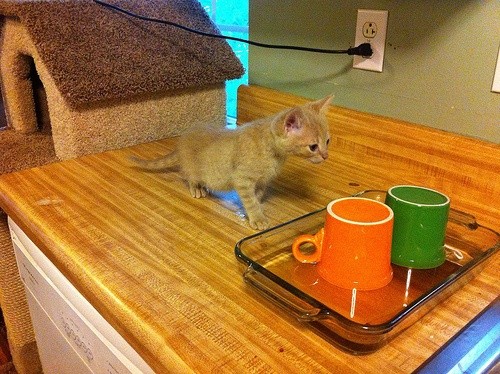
[292,196,394,290]
[386,184,450,269]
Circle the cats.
[121,94,334,230]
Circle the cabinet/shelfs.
[0,121,499,374]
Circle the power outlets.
[352,8,389,73]
